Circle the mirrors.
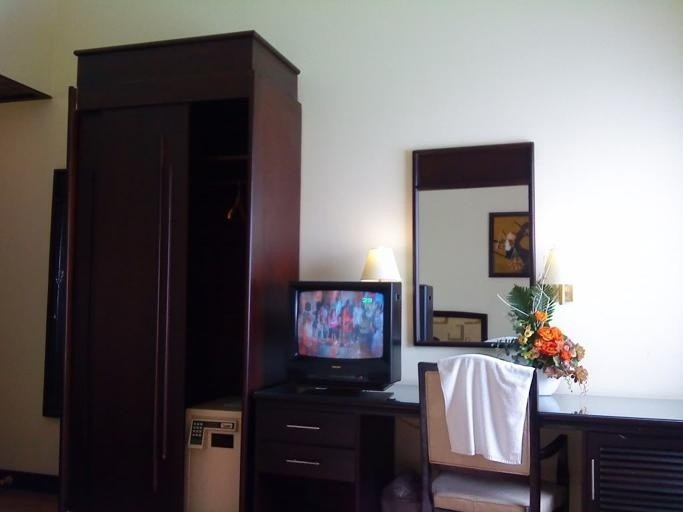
[412,141,536,347]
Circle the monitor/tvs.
[288,280,402,402]
[420,284,433,341]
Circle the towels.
[437,354,536,464]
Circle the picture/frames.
[488,212,532,276]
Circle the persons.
[297,299,384,356]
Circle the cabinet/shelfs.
[59,30,300,512]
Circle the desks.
[247,386,682,511]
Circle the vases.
[537,366,562,396]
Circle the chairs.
[419,353,570,512]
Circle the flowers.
[491,254,588,399]
